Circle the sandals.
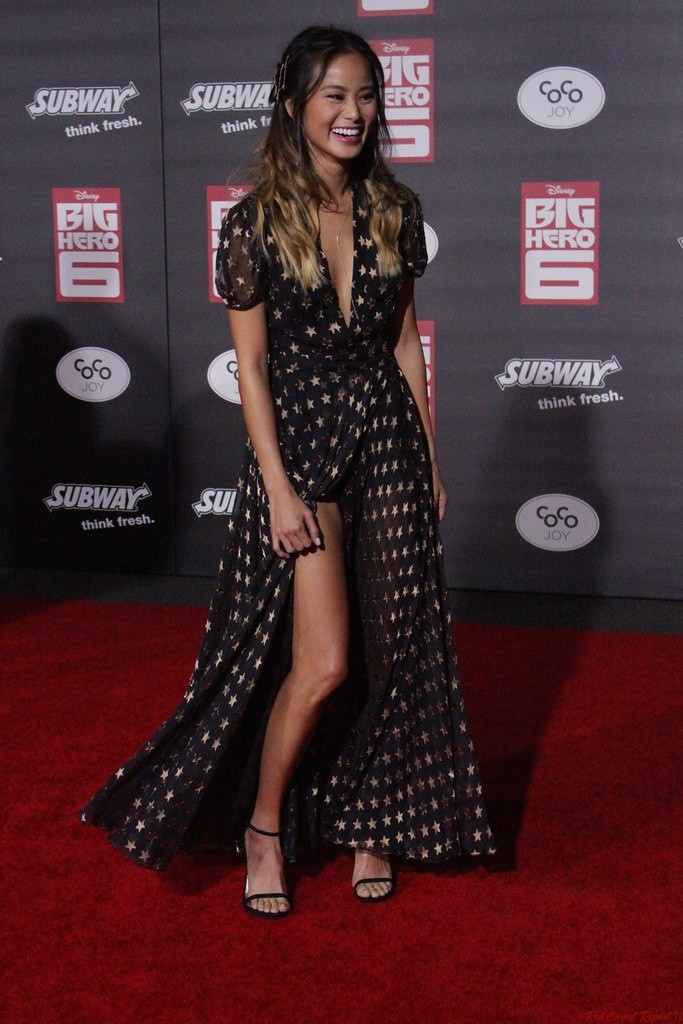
[351,850,395,901]
[242,824,293,918]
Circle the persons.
[215,25,448,919]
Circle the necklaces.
[319,192,353,242]
